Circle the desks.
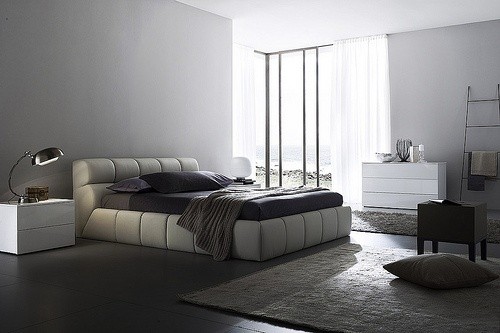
[417,200,488,262]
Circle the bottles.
[419,145,424,162]
[396,138,413,162]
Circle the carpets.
[180,242,500,333]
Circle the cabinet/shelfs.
[362,162,447,209]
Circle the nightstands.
[0,198,76,254]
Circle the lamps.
[230,157,252,182]
[7,147,64,204]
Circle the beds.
[73,158,352,262]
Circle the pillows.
[106,171,233,194]
[383,252,500,290]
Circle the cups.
[410,146,419,163]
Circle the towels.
[467,151,485,191]
[470,151,498,177]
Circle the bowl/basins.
[376,152,399,162]
